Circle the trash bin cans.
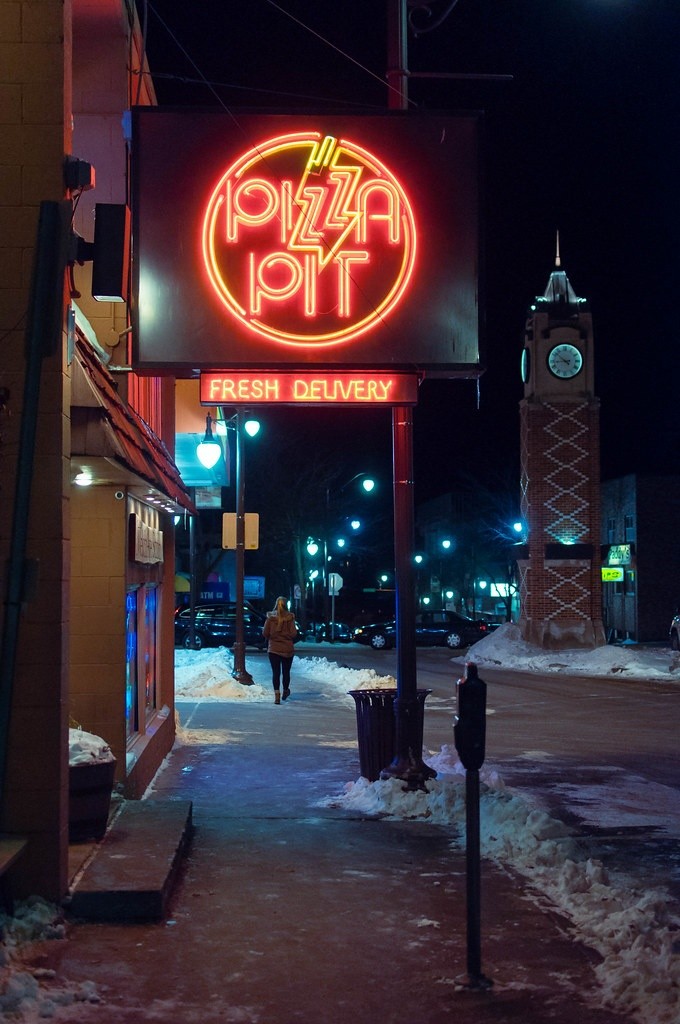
[69,759,118,846]
[348,688,434,782]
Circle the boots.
[282,689,291,700]
[273,690,281,704]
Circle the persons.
[262,595,298,704]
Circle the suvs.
[173,603,306,650]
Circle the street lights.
[322,470,375,643]
[195,411,261,686]
[306,536,346,619]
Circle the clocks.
[545,343,584,381]
[521,346,530,384]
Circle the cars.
[357,608,488,650]
[315,622,355,644]
